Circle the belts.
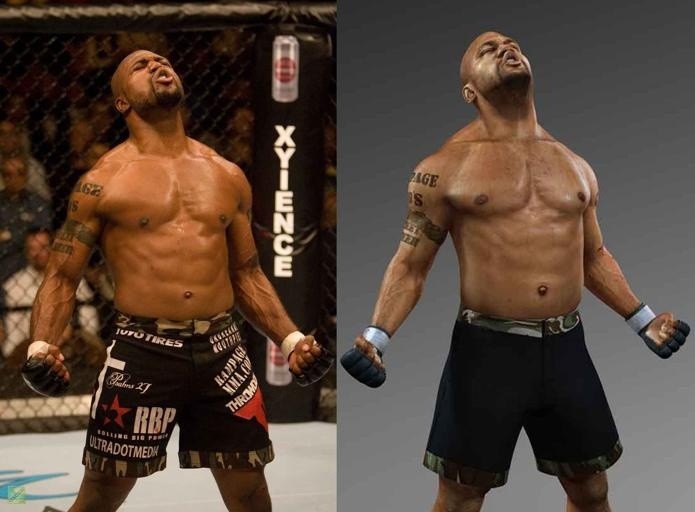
[112,313,236,334]
[461,305,588,338]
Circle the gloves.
[641,313,690,363]
[338,341,388,390]
[16,351,74,400]
[291,345,334,392]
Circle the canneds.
[266,337,293,387]
[271,34,300,103]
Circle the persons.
[338,30,692,510]
[19,49,335,511]
[0,3,334,400]
